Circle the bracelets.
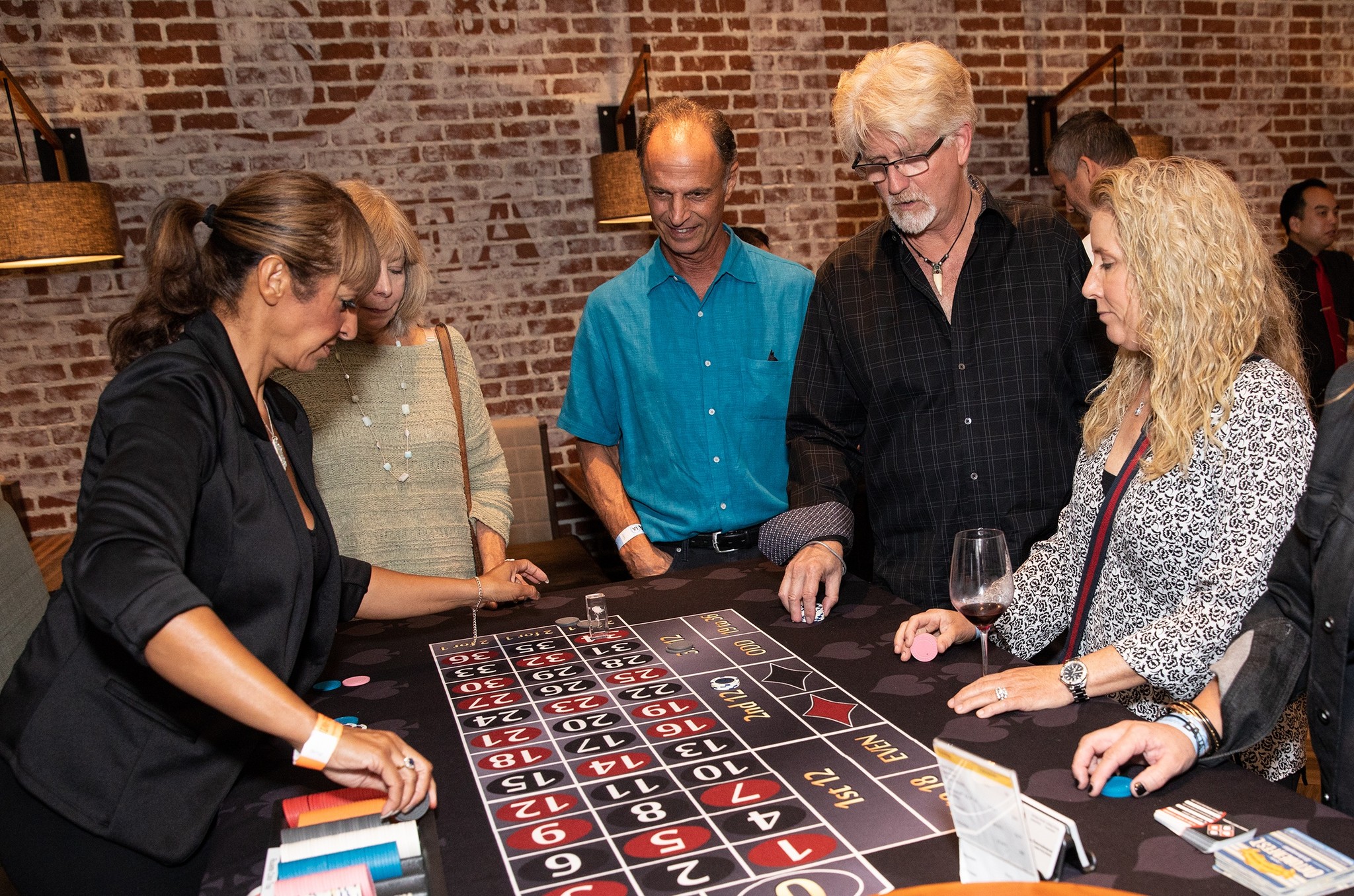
[974,626,981,642]
[291,712,344,771]
[798,540,847,576]
[1153,697,1222,758]
[468,576,482,646]
[615,523,645,551]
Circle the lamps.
[0,59,126,271]
[1025,45,1171,177]
[590,43,652,224]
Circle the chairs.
[1,492,50,699]
[492,416,604,589]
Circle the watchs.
[1058,654,1090,703]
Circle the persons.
[0,174,549,896]
[755,41,1123,629]
[553,96,819,578]
[1044,111,1140,266]
[1068,361,1352,816]
[268,177,526,616]
[1260,179,1354,418]
[892,155,1315,719]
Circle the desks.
[196,558,1354,896]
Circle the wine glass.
[949,529,1015,677]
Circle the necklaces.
[902,187,973,295]
[332,334,412,483]
[1136,381,1155,415]
[257,398,288,472]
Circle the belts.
[653,521,760,553]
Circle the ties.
[1312,254,1348,370]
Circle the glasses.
[851,133,948,182]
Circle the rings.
[397,756,416,771]
[995,686,1007,701]
